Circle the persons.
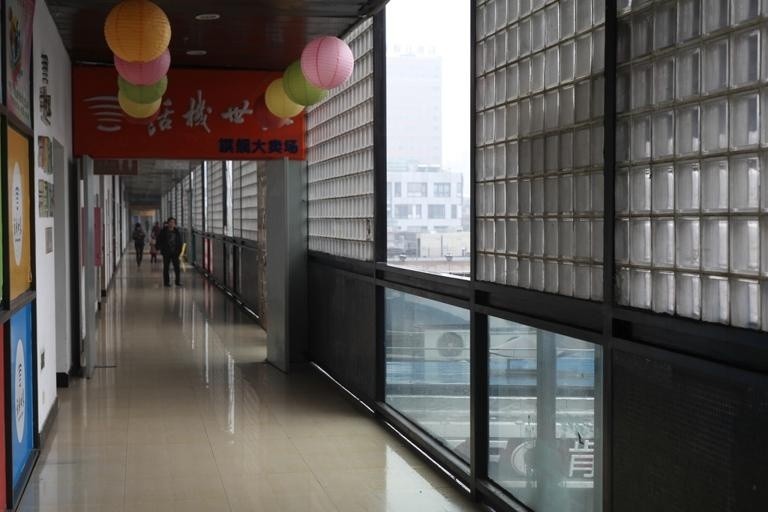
[132,217,182,287]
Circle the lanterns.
[264,35,355,119]
[104,0,172,118]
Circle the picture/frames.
[3,1,41,512]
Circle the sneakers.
[164,282,182,287]
